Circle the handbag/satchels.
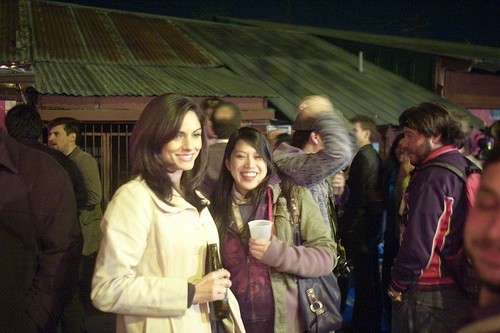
[297,270,345,332]
[211,314,240,333]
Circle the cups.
[248,220,273,242]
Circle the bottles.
[208,243,229,321]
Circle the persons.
[269,94,500,333]
[0,102,107,333]
[206,126,343,333]
[91,93,246,333]
